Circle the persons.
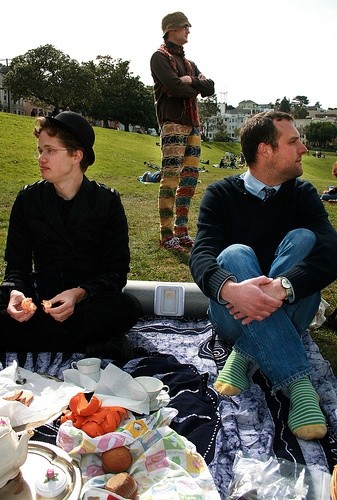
[150,11,215,255]
[0,112,142,360]
[138,149,337,201]
[187,110,337,440]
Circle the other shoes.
[160,236,195,253]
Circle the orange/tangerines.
[42,300,52,309]
[21,298,37,312]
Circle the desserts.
[105,472,138,500]
[101,447,132,474]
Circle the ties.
[262,186,276,201]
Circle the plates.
[80,473,116,500]
[149,390,170,411]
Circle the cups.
[36,468,68,498]
[70,358,101,383]
[130,376,170,402]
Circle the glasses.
[38,147,68,157]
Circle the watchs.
[278,275,293,301]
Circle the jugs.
[0,417,34,489]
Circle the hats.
[162,12,191,38]
[45,111,96,166]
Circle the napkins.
[0,360,86,427]
[63,364,150,415]
